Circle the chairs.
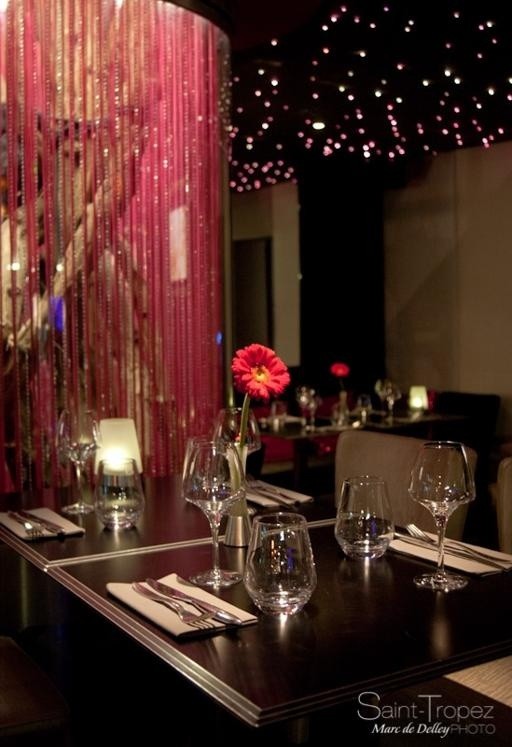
[334,430,477,541]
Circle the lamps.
[408,386,430,420]
[95,418,143,496]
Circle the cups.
[297,385,319,432]
[241,512,318,616]
[91,457,147,530]
[333,477,396,561]
[180,436,248,586]
[52,406,99,517]
[406,440,478,594]
[15,506,67,537]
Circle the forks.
[405,523,511,565]
[247,487,290,508]
[5,509,46,540]
[128,580,217,624]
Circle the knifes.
[253,481,299,503]
[145,576,242,626]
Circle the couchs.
[237,397,357,463]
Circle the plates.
[247,479,314,507]
[106,573,258,637]
[0,507,85,541]
